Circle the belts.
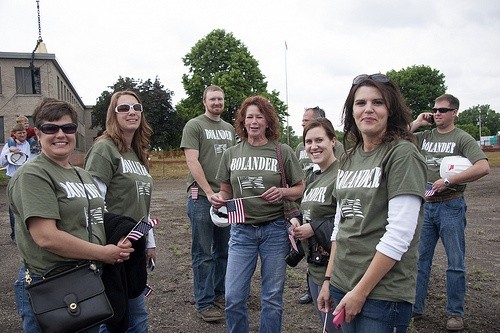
[426,192,464,202]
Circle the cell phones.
[332,307,345,330]
[149,258,155,271]
[429,115,434,124]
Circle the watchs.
[444,178,450,186]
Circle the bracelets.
[324,277,330,280]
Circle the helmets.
[8,152,29,166]
[210,204,231,227]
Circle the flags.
[128,218,158,241]
[227,198,245,224]
[143,285,152,297]
[425,183,435,196]
[289,228,299,253]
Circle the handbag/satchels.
[25,262,114,333]
[285,239,305,267]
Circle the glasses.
[432,107,458,113]
[353,74,389,85]
[39,123,77,134]
[115,103,143,113]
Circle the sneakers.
[412,312,425,319]
[197,307,222,321]
[300,291,313,304]
[213,298,225,309]
[446,316,464,330]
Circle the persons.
[6,97,157,333]
[317,73,428,333]
[7,114,40,152]
[179,85,242,321]
[210,95,306,333]
[404,94,490,330]
[295,106,345,304]
[288,117,340,326]
[83,90,156,333]
[0,124,40,242]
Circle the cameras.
[307,252,327,267]
[284,235,304,267]
